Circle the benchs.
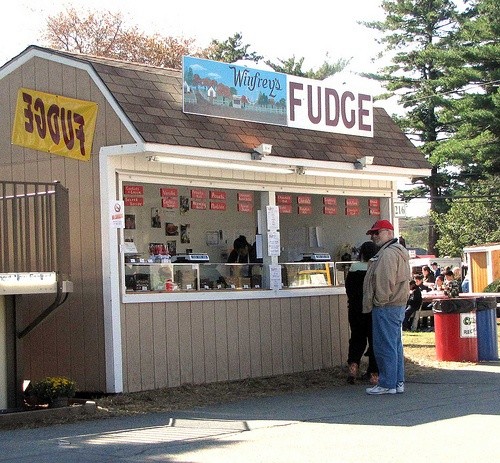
[405,310,434,330]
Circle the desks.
[410,289,500,310]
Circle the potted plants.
[31,375,80,407]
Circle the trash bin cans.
[431,297,479,364]
[458,292,499,363]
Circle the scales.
[294,252,332,262]
[170,252,210,264]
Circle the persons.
[227,234,258,287]
[344,241,378,385]
[361,220,410,393]
[401,261,470,330]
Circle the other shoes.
[370,373,378,383]
[428,327,434,331]
[348,363,357,384]
[423,328,428,332]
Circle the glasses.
[371,229,388,235]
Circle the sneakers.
[366,384,397,394]
[396,381,404,392]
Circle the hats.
[431,262,437,266]
[366,220,394,236]
